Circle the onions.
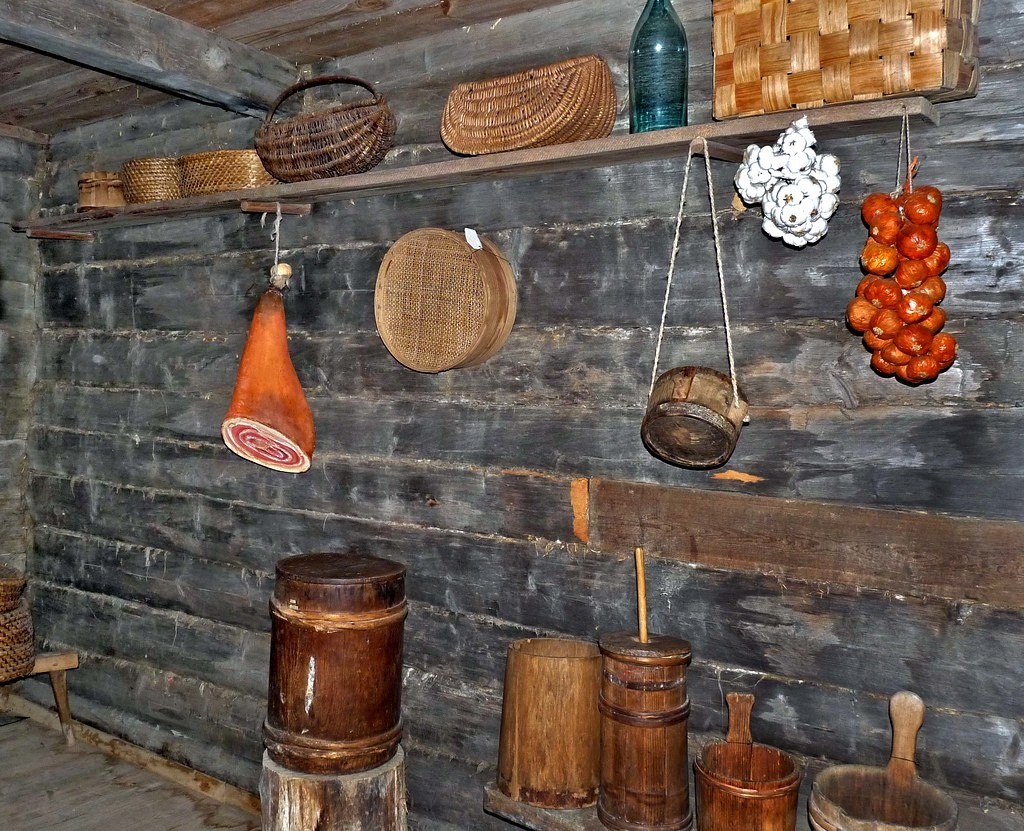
[846,186,958,385]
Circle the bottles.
[628,0,689,135]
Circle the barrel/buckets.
[640,366,749,471]
[496,636,603,810]
[598,634,694,831]
[807,689,960,831]
[264,555,409,775]
[693,691,803,831]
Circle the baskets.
[0,597,36,683]
[712,0,981,119]
[0,569,25,611]
[439,55,617,155]
[254,75,394,182]
[175,149,271,199]
[120,156,181,202]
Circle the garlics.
[733,117,843,247]
